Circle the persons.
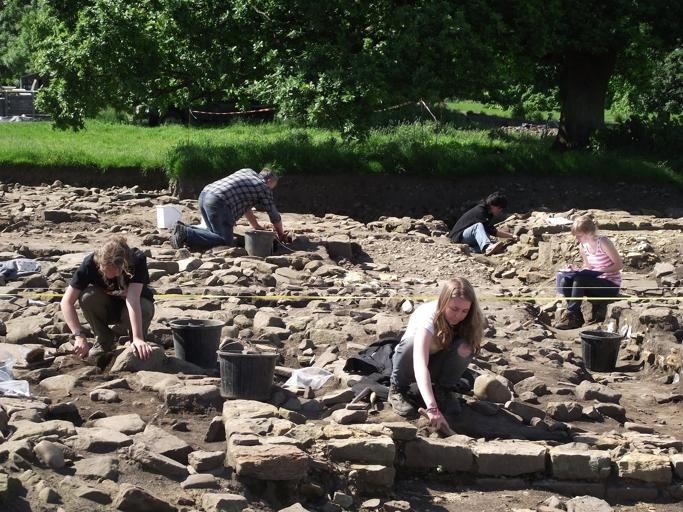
[386,277,487,433]
[61,235,155,361]
[449,192,519,255]
[171,167,288,249]
[554,213,624,329]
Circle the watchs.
[72,329,83,337]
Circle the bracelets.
[425,406,441,412]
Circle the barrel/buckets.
[156,205,183,229]
[244,228,274,257]
[216,349,280,401]
[169,319,225,370]
[579,330,622,373]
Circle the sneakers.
[387,392,414,417]
[554,313,578,330]
[552,312,584,327]
[173,224,186,249]
[170,221,185,248]
[88,333,114,356]
[439,391,461,414]
[485,241,502,255]
